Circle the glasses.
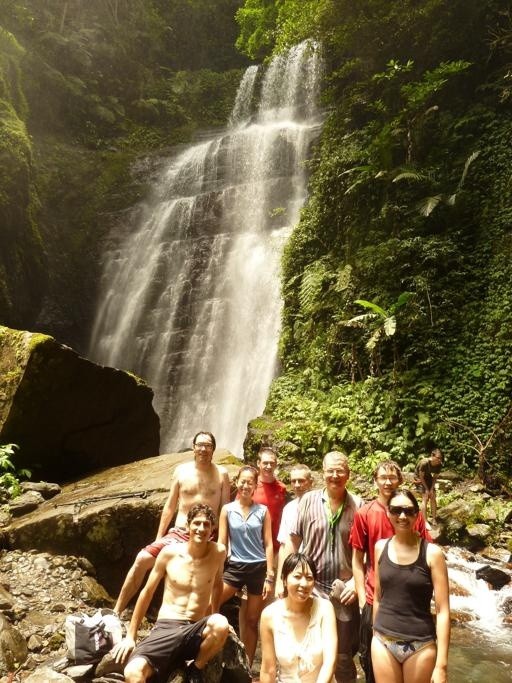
[388,505,417,516]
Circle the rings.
[350,590,354,593]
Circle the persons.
[110,502,228,683]
[290,451,368,683]
[348,461,434,683]
[218,465,275,678]
[112,432,230,619]
[275,463,314,600]
[369,488,451,683]
[234,447,287,642]
[259,553,339,683]
[413,449,443,531]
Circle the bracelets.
[264,570,274,583]
